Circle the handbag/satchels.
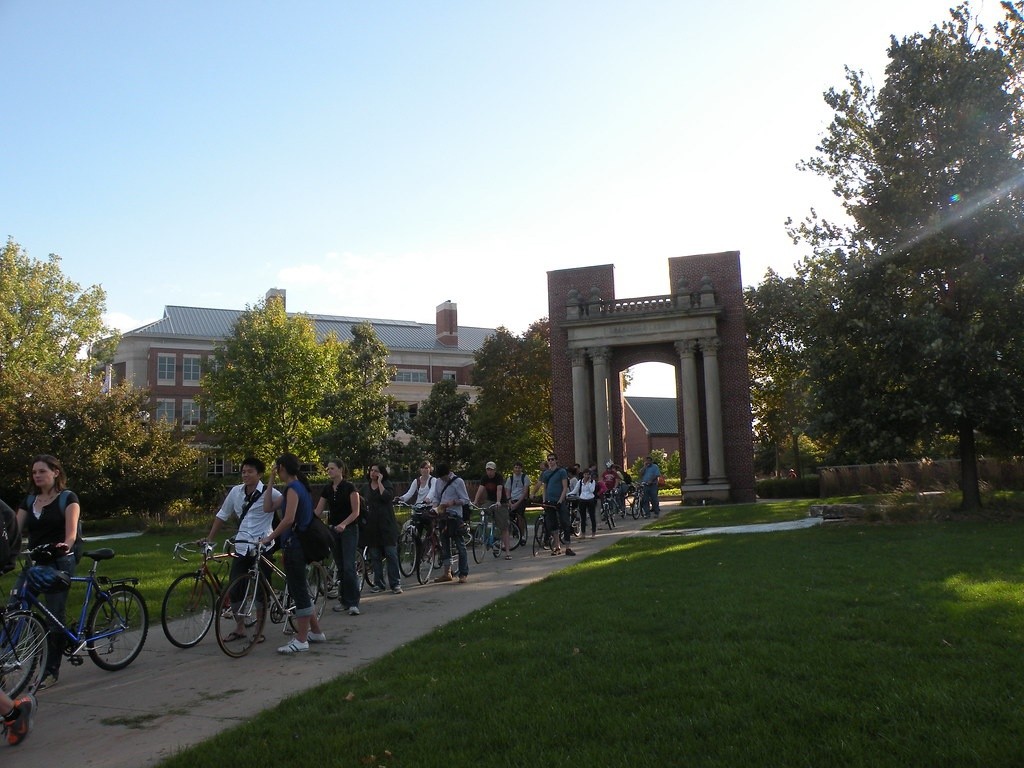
[299,517,336,563]
[269,518,282,554]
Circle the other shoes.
[505,555,512,560]
[458,575,467,583]
[434,574,453,582]
[551,547,562,555]
[575,534,585,539]
[566,549,576,556]
[592,532,595,539]
[520,539,527,546]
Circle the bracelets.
[453,500,456,506]
[508,498,511,501]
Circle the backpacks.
[27,490,88,568]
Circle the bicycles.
[531,482,654,557]
[215,538,328,659]
[161,540,259,648]
[392,500,444,577]
[0,609,52,723]
[307,547,366,605]
[412,508,460,585]
[470,501,502,564]
[0,543,150,724]
[490,497,529,551]
[317,544,388,600]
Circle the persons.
[194,457,283,645]
[11,452,81,674]
[474,452,660,560]
[398,460,443,567]
[430,461,473,584]
[313,457,362,615]
[357,462,404,595]
[0,681,37,748]
[261,454,325,653]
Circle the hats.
[435,461,449,478]
[486,461,496,470]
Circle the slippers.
[250,634,265,644]
[222,632,247,642]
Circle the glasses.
[547,458,556,461]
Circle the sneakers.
[307,631,326,642]
[277,638,309,653]
[392,586,403,593]
[333,603,345,611]
[29,671,59,691]
[348,606,360,615]
[370,586,387,592]
[1,693,36,745]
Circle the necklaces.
[37,494,57,502]
